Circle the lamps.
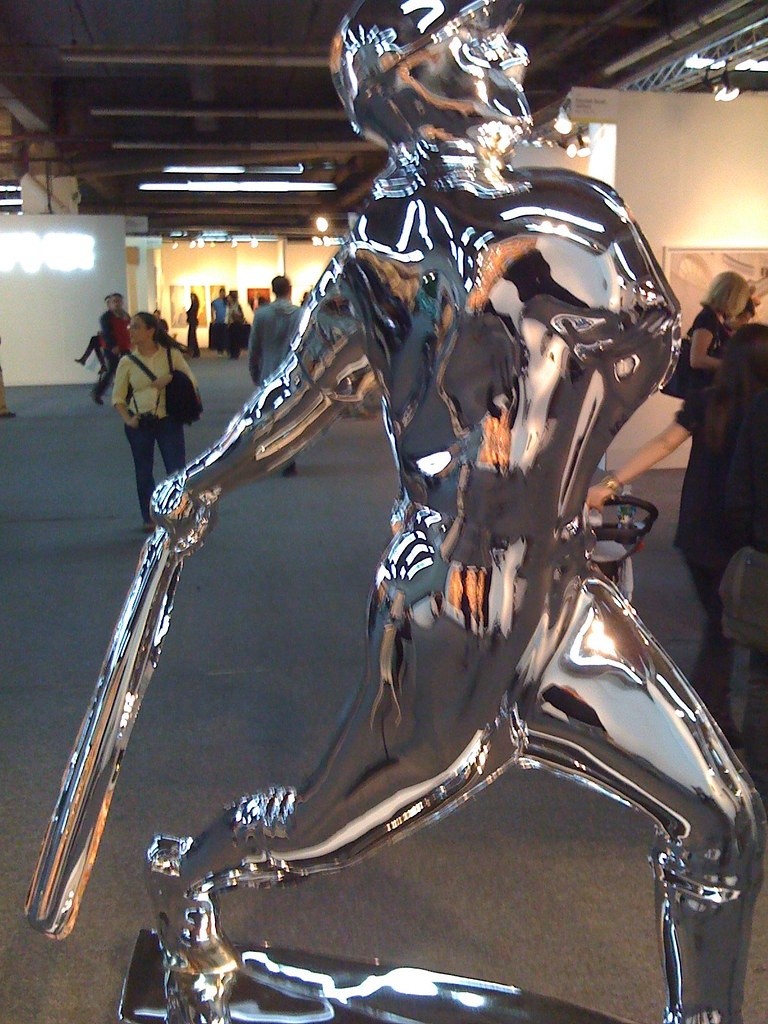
[249,233,258,247]
[553,104,591,158]
[230,235,238,248]
[701,67,741,104]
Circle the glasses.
[127,324,151,331]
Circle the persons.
[586,323,768,808]
[112,312,201,532]
[211,288,243,359]
[249,276,369,477]
[75,293,135,406]
[686,272,754,368]
[0,337,16,416]
[154,310,168,342]
[148,0,768,1024]
[187,293,200,358]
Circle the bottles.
[617,485,636,531]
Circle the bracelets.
[601,474,625,494]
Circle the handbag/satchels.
[661,321,720,399]
[718,546,768,648]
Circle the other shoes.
[99,368,107,374]
[90,390,103,404]
[143,521,155,532]
[713,702,745,748]
[1,411,16,419]
[75,358,85,365]
